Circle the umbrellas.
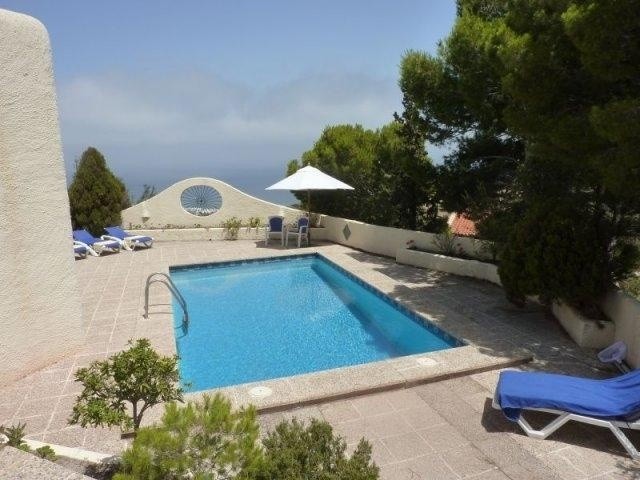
[264,161,355,224]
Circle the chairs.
[264,215,285,247]
[73,241,90,259]
[101,226,155,251]
[73,230,120,256]
[492,368,640,460]
[286,217,309,248]
